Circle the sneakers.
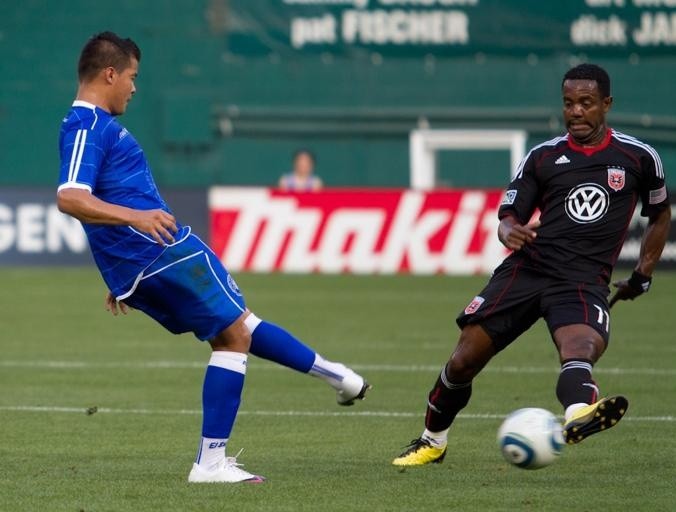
[187,456,264,485]
[391,439,449,465]
[561,395,629,445]
[335,368,372,406]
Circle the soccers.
[496,408,566,471]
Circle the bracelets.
[628,270,653,293]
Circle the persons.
[57,31,372,483]
[392,64,671,467]
[279,149,323,188]
